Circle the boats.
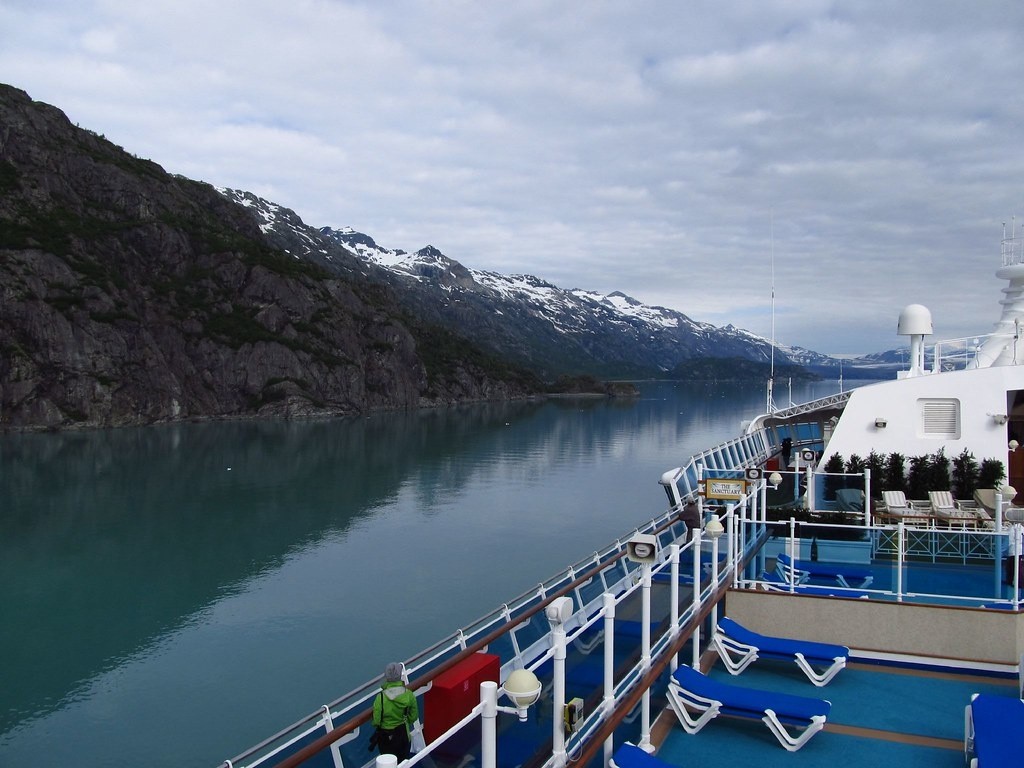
[217,212,1023,768]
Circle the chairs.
[929,487,977,530]
[835,488,876,521]
[571,552,874,768]
[979,589,1024,609]
[974,488,1019,528]
[882,487,928,523]
[965,686,1024,768]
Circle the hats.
[385,662,402,682]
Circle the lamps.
[1008,440,1019,452]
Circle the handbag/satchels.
[368,725,382,751]
[410,718,426,753]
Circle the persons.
[816,450,824,466]
[371,662,418,765]
[782,438,792,468]
